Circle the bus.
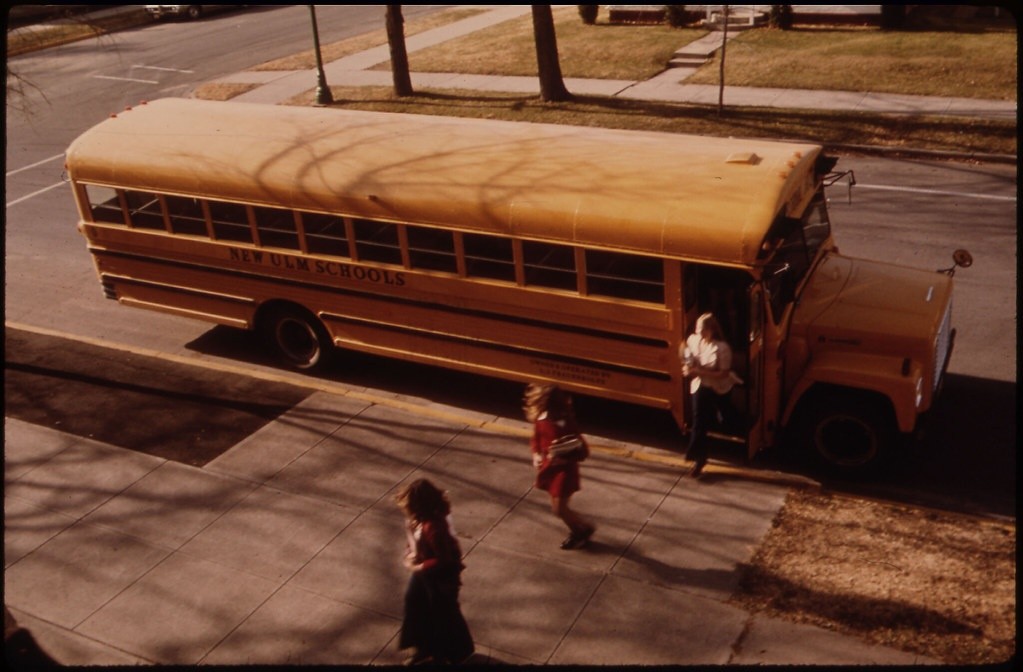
[65,95,976,476]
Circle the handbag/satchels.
[548,434,587,463]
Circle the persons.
[681,313,744,477]
[393,477,475,666]
[523,381,596,550]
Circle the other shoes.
[691,461,705,476]
[404,653,433,666]
[441,656,470,665]
[560,528,593,548]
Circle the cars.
[144,5,204,22]
